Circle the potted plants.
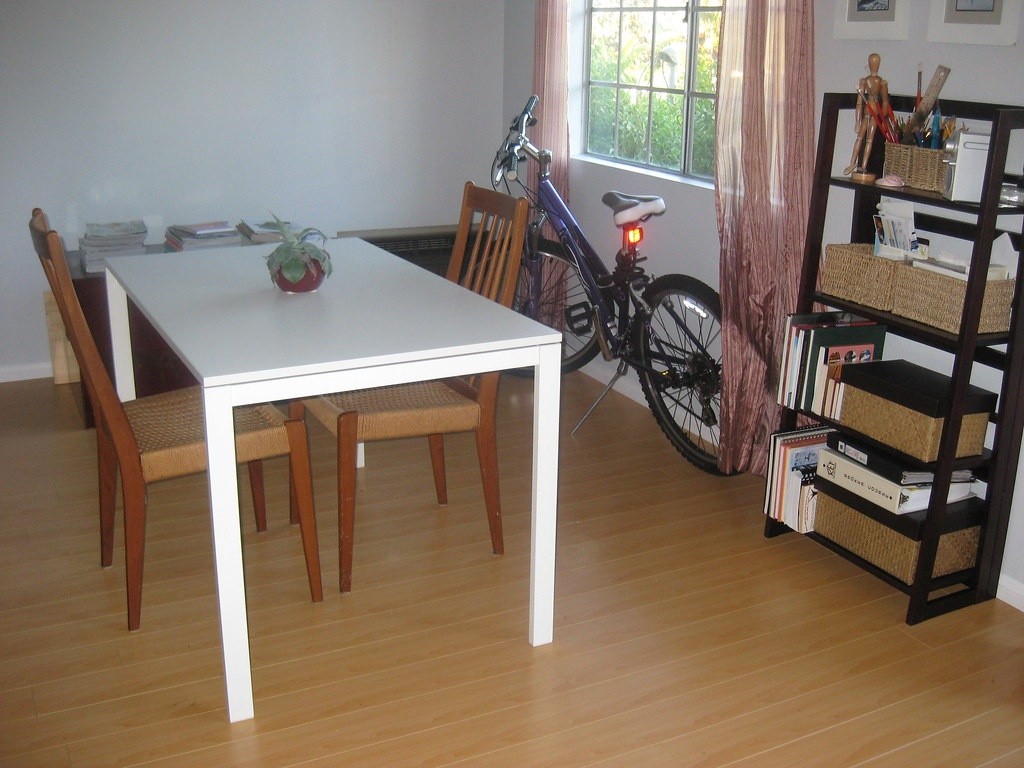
[265,212,331,291]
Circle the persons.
[843,54,889,174]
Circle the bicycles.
[440,94,748,478]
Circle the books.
[236,219,316,244]
[763,424,979,535]
[78,220,149,272]
[165,221,241,252]
[913,257,1008,281]
[777,311,888,420]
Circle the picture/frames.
[833,0,911,41]
[928,0,1018,46]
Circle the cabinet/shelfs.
[65,244,200,427]
[764,92,1024,626]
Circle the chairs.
[30,208,324,631]
[291,181,528,592]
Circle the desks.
[104,237,564,724]
